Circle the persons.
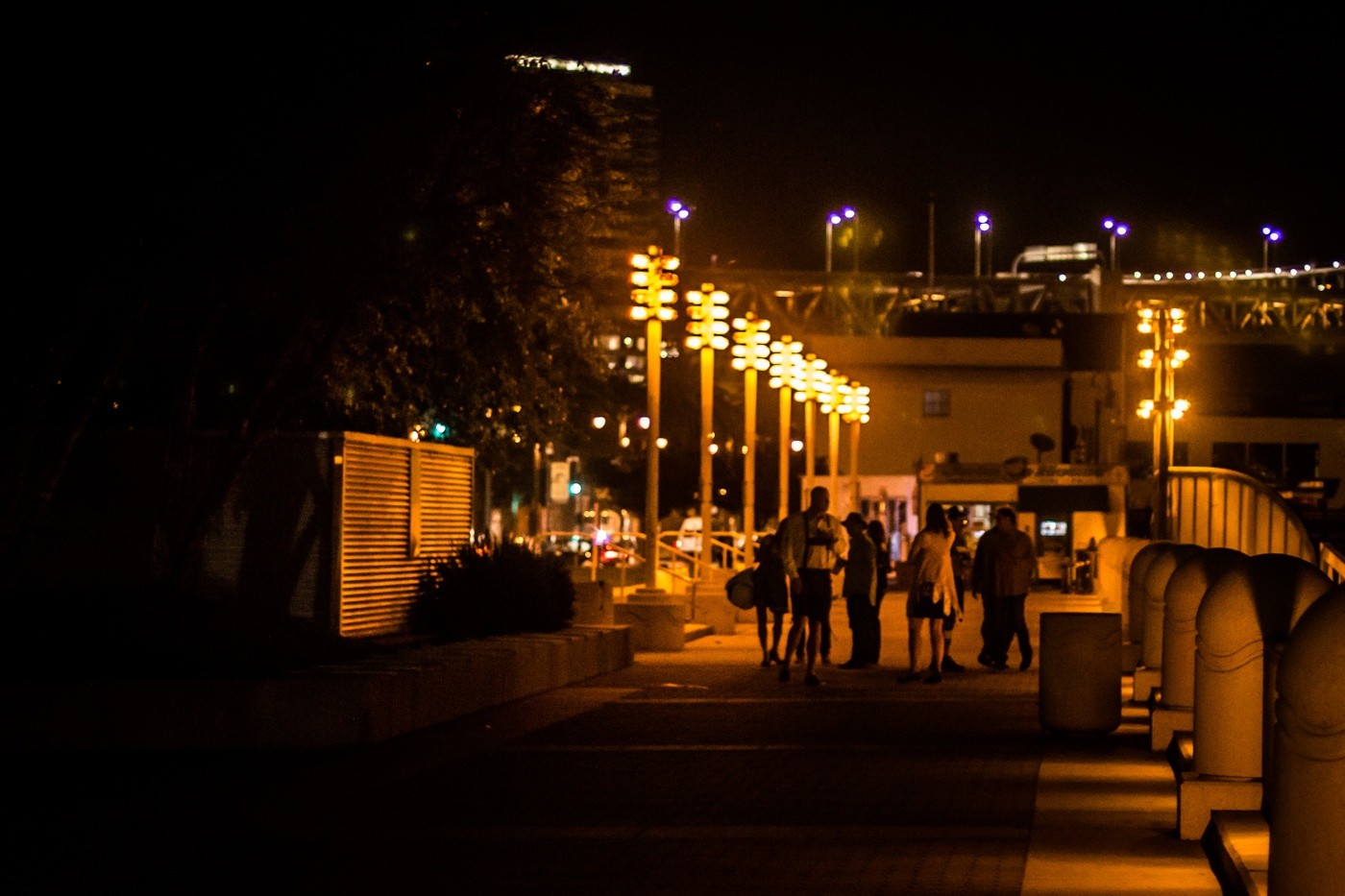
[971,505,1039,671]
[724,518,789,668]
[779,486,850,687]
[836,513,878,670]
[942,504,970,672]
[866,520,890,664]
[896,503,963,684]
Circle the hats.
[840,512,868,530]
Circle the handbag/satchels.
[725,567,757,609]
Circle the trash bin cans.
[1039,612,1124,735]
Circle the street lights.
[1135,305,1195,469]
[668,199,689,256]
[627,244,680,592]
[684,283,733,580]
[727,310,773,565]
[823,212,843,271]
[844,209,861,269]
[1261,226,1280,271]
[1104,220,1129,272]
[792,354,870,501]
[973,214,996,277]
[767,334,807,522]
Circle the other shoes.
[945,655,965,671]
[769,650,782,664]
[1019,651,1034,670]
[781,672,790,684]
[761,659,771,667]
[976,653,1010,670]
[837,660,863,669]
[807,674,824,684]
